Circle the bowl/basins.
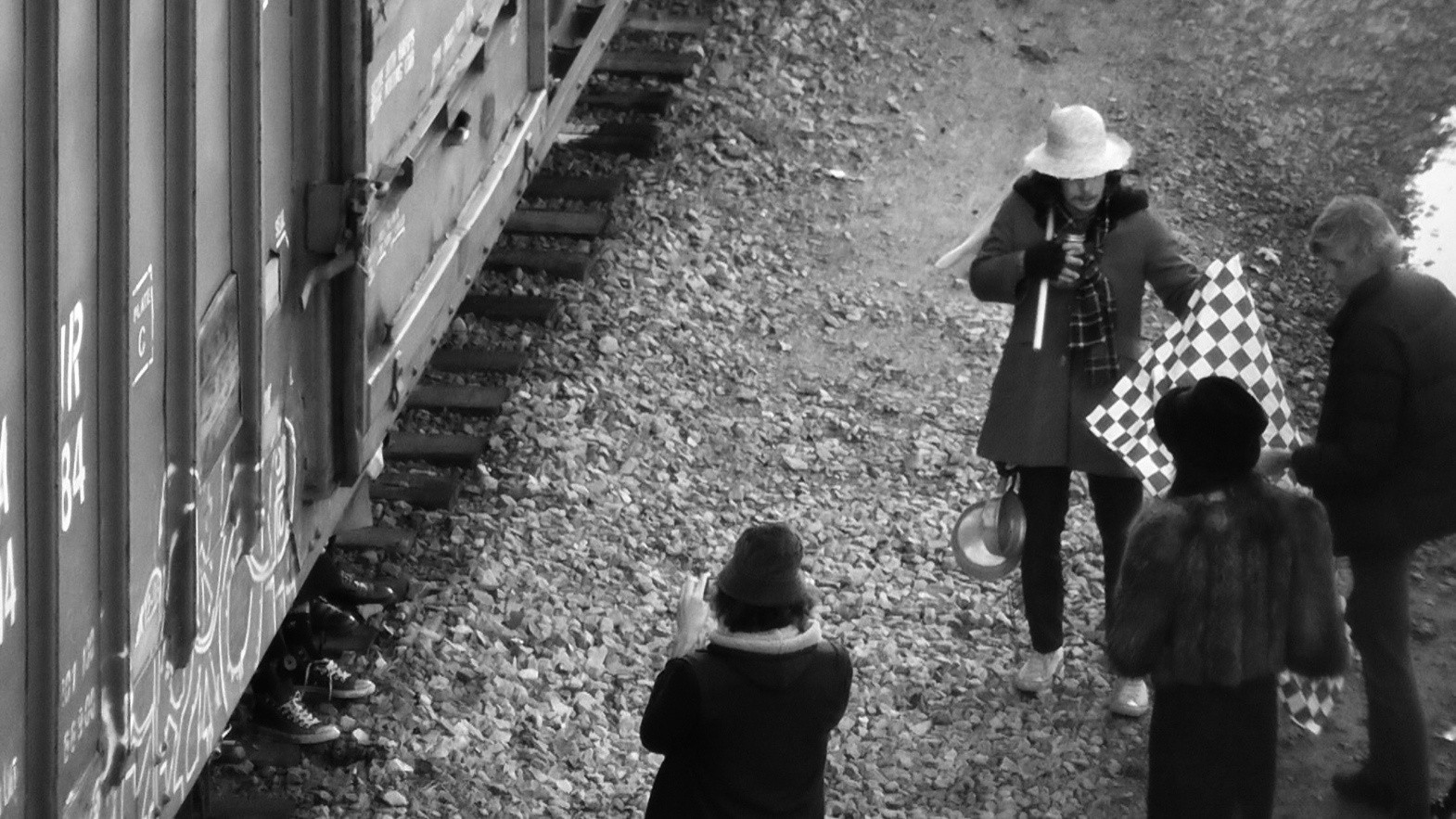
[978,489,1025,560]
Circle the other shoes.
[1331,763,1392,806]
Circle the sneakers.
[1110,675,1149,718]
[1017,645,1064,692]
[289,647,376,704]
[331,570,393,602]
[248,692,340,745]
[305,596,360,633]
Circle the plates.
[953,504,1023,581]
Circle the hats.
[1024,104,1132,181]
[715,523,806,607]
[1154,377,1267,476]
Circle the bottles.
[1061,229,1086,274]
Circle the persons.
[1105,376,1354,819]
[250,553,397,743]
[1256,196,1456,819]
[640,522,853,819]
[969,104,1204,717]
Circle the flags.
[1082,254,1346,735]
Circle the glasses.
[1308,245,1358,271]
